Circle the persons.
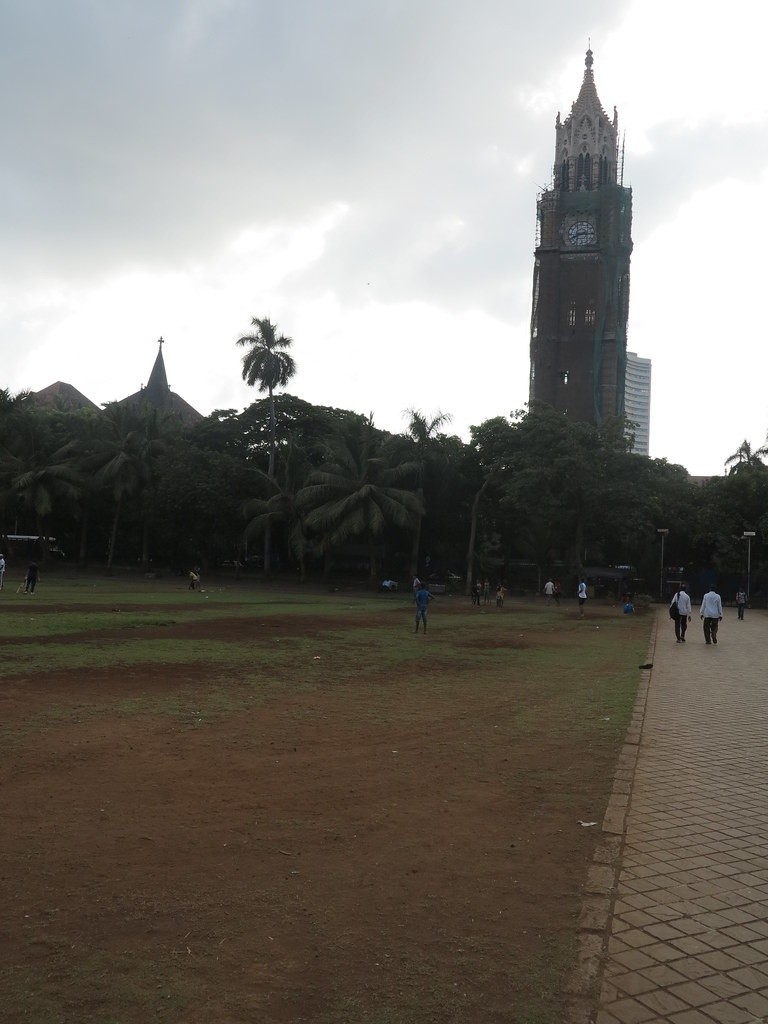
[699,584,723,644]
[413,582,435,634]
[472,574,563,608]
[381,578,393,594]
[411,574,422,603]
[22,558,40,595]
[735,587,747,620]
[0,554,5,589]
[576,578,588,617]
[669,584,692,642]
[187,571,199,591]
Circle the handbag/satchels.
[669,592,681,620]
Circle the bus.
[2,535,65,559]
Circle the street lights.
[657,528,669,602]
[744,532,755,601]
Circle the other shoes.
[681,636,685,641]
[23,591,27,594]
[677,639,680,643]
[31,592,35,594]
[713,639,717,643]
[706,641,711,644]
